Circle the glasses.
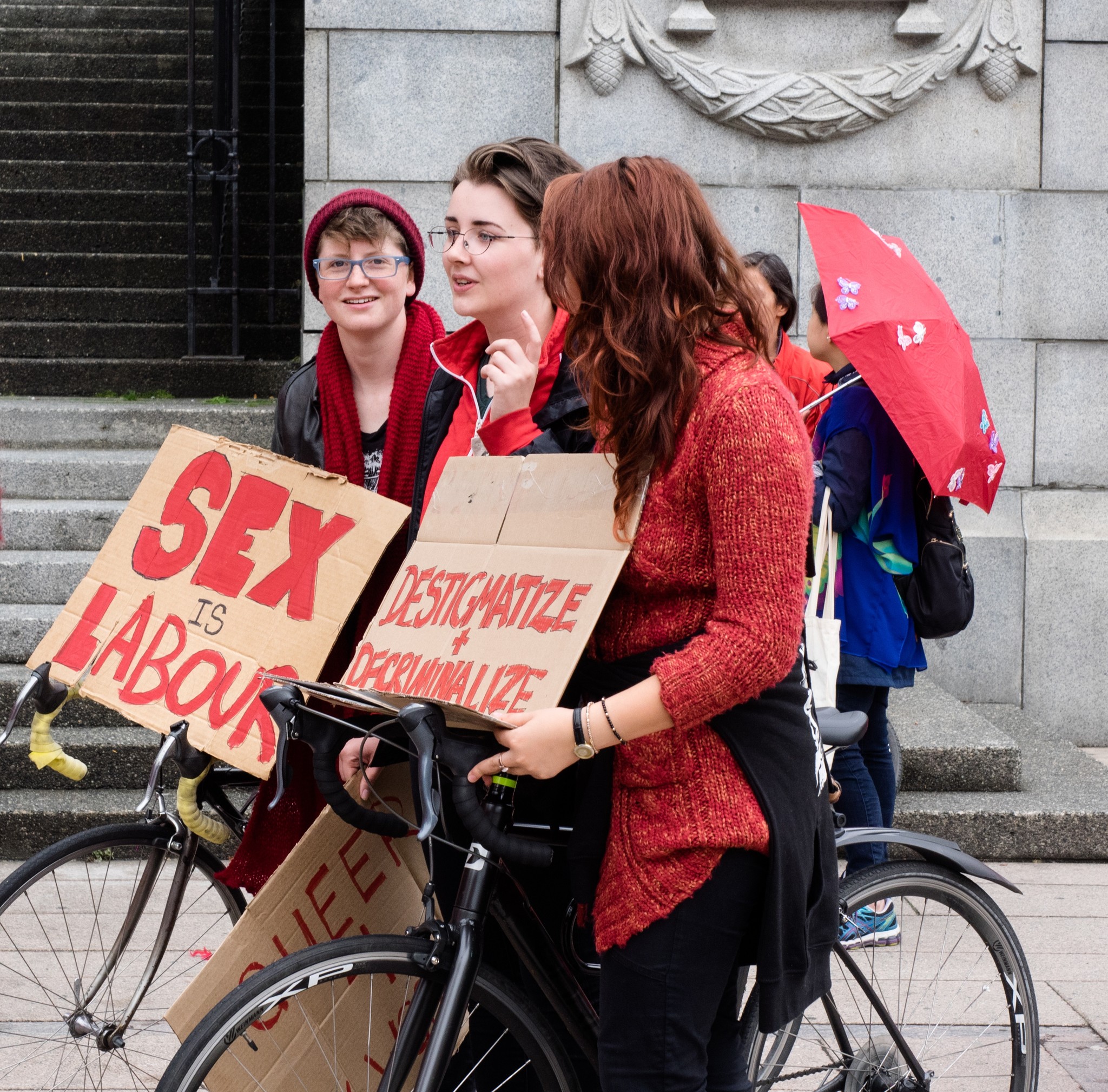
[313,256,411,280]
[427,226,541,256]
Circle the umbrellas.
[787,196,1009,519]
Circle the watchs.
[572,707,599,761]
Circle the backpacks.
[847,381,973,639]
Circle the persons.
[428,155,818,1092]
[330,132,651,1089]
[795,280,924,953]
[261,188,454,666]
[732,245,838,448]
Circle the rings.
[496,750,510,774]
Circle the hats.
[303,188,424,307]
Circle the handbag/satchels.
[802,613,842,772]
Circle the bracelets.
[597,694,631,749]
[584,701,604,755]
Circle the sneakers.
[832,897,901,951]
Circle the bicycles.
[152,679,1041,1092]
[0,661,262,1090]
